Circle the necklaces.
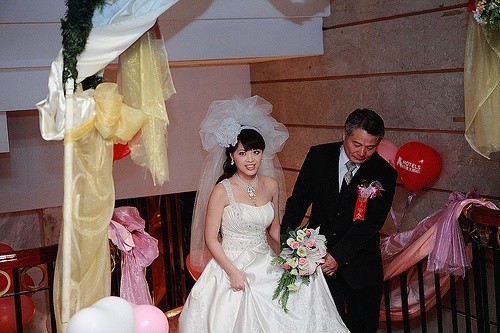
[233,172,259,200]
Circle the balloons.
[395,142,442,193]
[376,139,398,168]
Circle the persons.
[178,95,352,333]
[278,109,398,333]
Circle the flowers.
[356,179,384,199]
[215,117,242,148]
[472,0,500,39]
[269,223,329,313]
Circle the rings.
[237,288,240,291]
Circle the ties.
[340,160,357,194]
